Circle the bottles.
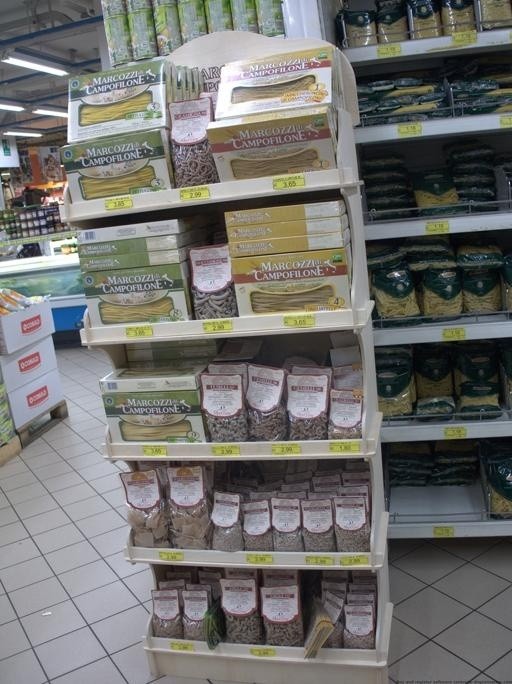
[100,0,284,66]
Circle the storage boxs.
[0,300,63,432]
